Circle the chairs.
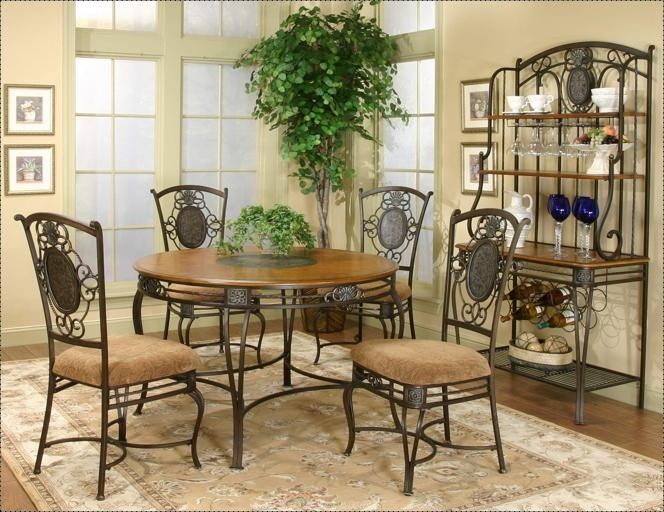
[150,184,266,368]
[343,208,530,497]
[14,212,204,500]
[313,186,432,367]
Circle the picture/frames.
[460,77,498,131]
[4,83,56,136]
[4,143,56,197]
[459,141,499,196]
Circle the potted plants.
[233,0,411,334]
[213,203,316,311]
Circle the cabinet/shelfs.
[456,41,655,424]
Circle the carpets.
[0,330,664,512]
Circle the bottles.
[537,309,583,329]
[502,281,544,301]
[526,286,575,309]
[500,301,547,323]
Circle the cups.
[507,122,526,157]
[545,193,570,259]
[558,124,576,160]
[523,123,544,156]
[505,95,528,111]
[571,196,598,262]
[542,126,559,157]
[527,94,553,111]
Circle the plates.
[503,111,522,116]
[572,143,634,174]
[528,110,550,114]
[523,108,551,114]
[500,109,528,114]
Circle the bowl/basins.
[590,87,625,94]
[590,93,627,114]
